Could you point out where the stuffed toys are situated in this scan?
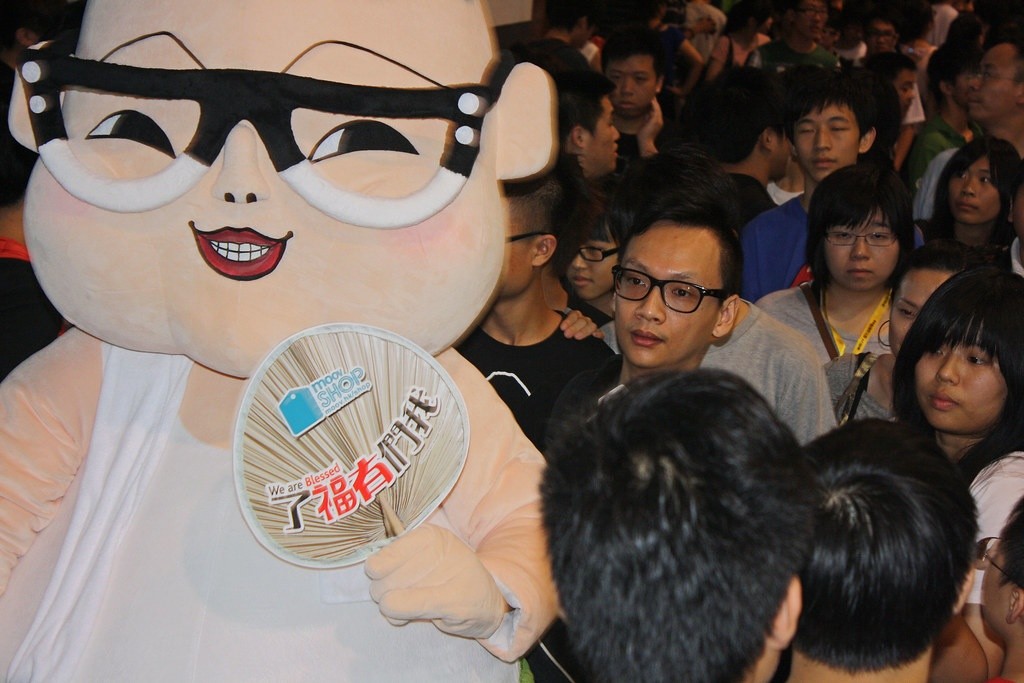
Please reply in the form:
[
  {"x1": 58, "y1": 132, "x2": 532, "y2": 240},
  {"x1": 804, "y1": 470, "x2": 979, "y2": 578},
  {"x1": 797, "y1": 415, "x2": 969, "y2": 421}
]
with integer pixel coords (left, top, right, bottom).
[{"x1": 0, "y1": 0, "x2": 562, "y2": 683}]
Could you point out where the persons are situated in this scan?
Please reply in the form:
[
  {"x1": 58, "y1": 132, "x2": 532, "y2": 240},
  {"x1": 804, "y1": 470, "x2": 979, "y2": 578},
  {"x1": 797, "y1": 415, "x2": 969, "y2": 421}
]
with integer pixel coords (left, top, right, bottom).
[
  {"x1": 455, "y1": 0, "x2": 1024, "y2": 683},
  {"x1": 0, "y1": 0, "x2": 88, "y2": 381}
]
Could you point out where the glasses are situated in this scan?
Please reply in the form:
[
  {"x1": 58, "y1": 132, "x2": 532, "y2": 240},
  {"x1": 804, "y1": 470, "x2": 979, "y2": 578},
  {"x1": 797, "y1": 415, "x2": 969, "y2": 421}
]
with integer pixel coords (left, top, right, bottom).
[
  {"x1": 968, "y1": 66, "x2": 1019, "y2": 82},
  {"x1": 564, "y1": 246, "x2": 619, "y2": 262},
  {"x1": 825, "y1": 231, "x2": 897, "y2": 247},
  {"x1": 611, "y1": 265, "x2": 725, "y2": 313},
  {"x1": 973, "y1": 536, "x2": 1018, "y2": 588},
  {"x1": 867, "y1": 29, "x2": 894, "y2": 40},
  {"x1": 794, "y1": 7, "x2": 828, "y2": 18}
]
